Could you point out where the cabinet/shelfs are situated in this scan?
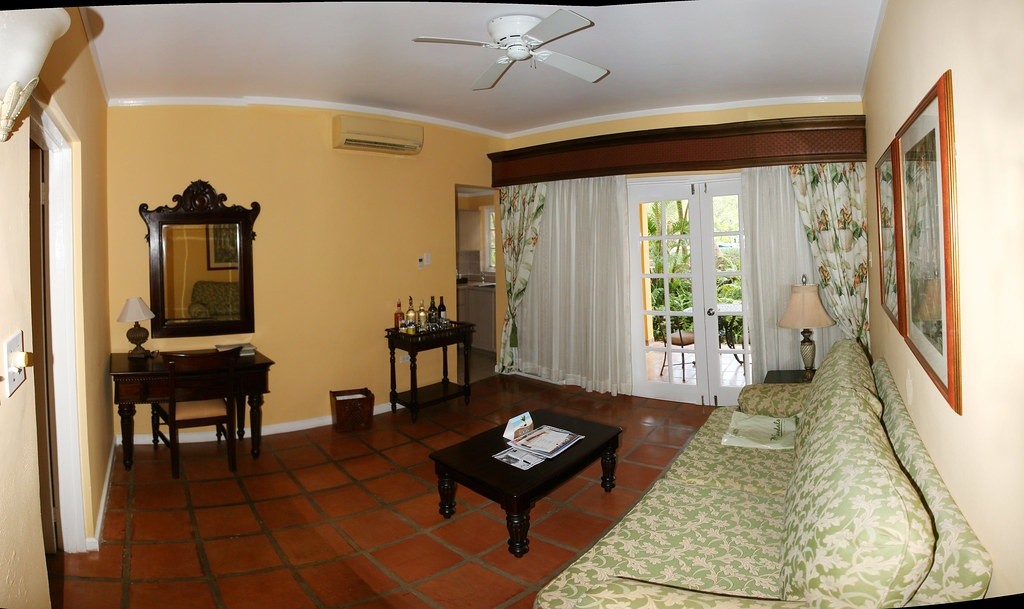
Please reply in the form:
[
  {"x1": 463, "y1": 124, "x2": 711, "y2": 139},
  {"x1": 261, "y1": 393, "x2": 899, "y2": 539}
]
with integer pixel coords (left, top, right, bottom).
[
  {"x1": 384, "y1": 319, "x2": 478, "y2": 424},
  {"x1": 456, "y1": 289, "x2": 495, "y2": 352}
]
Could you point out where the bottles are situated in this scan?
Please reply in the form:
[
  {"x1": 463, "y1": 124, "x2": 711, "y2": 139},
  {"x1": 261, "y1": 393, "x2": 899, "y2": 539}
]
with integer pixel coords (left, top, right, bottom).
[
  {"x1": 405, "y1": 295, "x2": 416, "y2": 326},
  {"x1": 400, "y1": 320, "x2": 407, "y2": 333},
  {"x1": 427, "y1": 296, "x2": 438, "y2": 324},
  {"x1": 407, "y1": 320, "x2": 415, "y2": 335},
  {"x1": 438, "y1": 296, "x2": 446, "y2": 322},
  {"x1": 416, "y1": 300, "x2": 427, "y2": 326},
  {"x1": 394, "y1": 299, "x2": 404, "y2": 328}
]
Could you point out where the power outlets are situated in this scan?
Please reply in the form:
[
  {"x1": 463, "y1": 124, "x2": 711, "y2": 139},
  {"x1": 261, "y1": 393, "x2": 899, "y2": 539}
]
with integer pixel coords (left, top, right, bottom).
[{"x1": 4, "y1": 328, "x2": 26, "y2": 399}]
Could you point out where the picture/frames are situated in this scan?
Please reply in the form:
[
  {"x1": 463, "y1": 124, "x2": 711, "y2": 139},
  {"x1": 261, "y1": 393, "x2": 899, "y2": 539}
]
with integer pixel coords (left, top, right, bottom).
[
  {"x1": 875, "y1": 69, "x2": 962, "y2": 416},
  {"x1": 206, "y1": 222, "x2": 240, "y2": 271}
]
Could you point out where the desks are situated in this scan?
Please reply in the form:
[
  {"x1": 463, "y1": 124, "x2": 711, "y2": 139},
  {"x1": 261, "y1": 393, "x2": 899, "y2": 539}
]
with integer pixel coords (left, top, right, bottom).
[
  {"x1": 109, "y1": 348, "x2": 276, "y2": 472},
  {"x1": 683, "y1": 302, "x2": 744, "y2": 365}
]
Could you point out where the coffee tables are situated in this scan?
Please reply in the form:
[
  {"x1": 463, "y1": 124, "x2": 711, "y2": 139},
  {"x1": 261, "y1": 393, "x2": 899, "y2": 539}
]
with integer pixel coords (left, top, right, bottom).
[
  {"x1": 427, "y1": 408, "x2": 624, "y2": 559},
  {"x1": 764, "y1": 368, "x2": 817, "y2": 382}
]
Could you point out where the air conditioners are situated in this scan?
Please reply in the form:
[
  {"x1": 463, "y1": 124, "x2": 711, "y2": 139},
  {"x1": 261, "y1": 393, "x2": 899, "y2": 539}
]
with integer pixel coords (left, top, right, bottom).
[{"x1": 330, "y1": 114, "x2": 424, "y2": 156}]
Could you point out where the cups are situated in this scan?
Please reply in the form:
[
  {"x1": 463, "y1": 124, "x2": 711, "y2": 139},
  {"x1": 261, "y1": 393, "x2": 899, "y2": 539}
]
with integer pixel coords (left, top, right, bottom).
[{"x1": 419, "y1": 319, "x2": 450, "y2": 334}]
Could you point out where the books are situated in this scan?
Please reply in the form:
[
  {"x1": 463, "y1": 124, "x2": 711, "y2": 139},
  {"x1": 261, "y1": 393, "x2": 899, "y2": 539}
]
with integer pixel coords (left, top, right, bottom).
[{"x1": 507, "y1": 425, "x2": 586, "y2": 459}]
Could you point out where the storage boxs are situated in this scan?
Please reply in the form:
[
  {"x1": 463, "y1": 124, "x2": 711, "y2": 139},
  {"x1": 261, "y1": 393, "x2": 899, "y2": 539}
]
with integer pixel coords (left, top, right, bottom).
[{"x1": 328, "y1": 385, "x2": 375, "y2": 433}]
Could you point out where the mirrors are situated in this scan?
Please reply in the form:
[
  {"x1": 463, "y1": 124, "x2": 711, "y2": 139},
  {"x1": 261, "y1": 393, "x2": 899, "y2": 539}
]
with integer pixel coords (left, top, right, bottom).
[{"x1": 138, "y1": 178, "x2": 262, "y2": 340}]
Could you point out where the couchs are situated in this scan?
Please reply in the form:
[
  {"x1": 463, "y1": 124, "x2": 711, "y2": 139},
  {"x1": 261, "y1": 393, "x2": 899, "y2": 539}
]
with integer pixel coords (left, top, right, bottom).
[
  {"x1": 188, "y1": 280, "x2": 241, "y2": 322},
  {"x1": 532, "y1": 336, "x2": 992, "y2": 609}
]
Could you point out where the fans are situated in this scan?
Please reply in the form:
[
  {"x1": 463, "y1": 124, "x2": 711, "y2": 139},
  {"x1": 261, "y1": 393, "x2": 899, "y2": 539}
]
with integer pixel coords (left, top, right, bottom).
[{"x1": 412, "y1": 7, "x2": 609, "y2": 91}]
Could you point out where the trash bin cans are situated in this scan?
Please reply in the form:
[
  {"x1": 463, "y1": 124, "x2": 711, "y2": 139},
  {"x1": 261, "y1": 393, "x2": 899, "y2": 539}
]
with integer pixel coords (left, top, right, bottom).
[{"x1": 330, "y1": 387, "x2": 376, "y2": 434}]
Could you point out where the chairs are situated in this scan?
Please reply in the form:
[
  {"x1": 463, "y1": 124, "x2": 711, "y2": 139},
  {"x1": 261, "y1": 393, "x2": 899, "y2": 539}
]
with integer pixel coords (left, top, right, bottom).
[
  {"x1": 150, "y1": 345, "x2": 246, "y2": 479},
  {"x1": 657, "y1": 314, "x2": 695, "y2": 382}
]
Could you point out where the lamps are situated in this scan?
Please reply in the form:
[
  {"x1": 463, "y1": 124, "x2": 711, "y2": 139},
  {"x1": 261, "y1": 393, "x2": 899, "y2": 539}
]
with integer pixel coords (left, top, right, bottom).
[
  {"x1": 117, "y1": 297, "x2": 155, "y2": 358},
  {"x1": 777, "y1": 274, "x2": 837, "y2": 381},
  {"x1": 1, "y1": 7, "x2": 73, "y2": 144}
]
